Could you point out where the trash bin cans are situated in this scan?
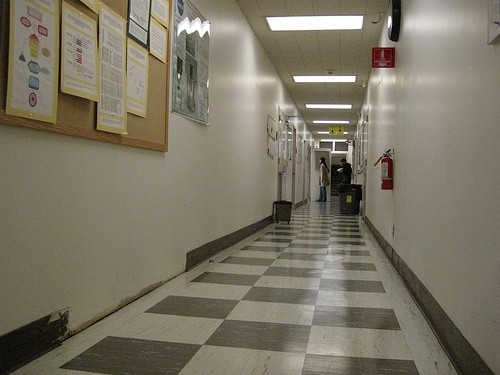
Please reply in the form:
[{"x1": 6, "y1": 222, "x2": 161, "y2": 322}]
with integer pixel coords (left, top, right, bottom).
[
  {"x1": 272, "y1": 200, "x2": 292, "y2": 225},
  {"x1": 338, "y1": 183, "x2": 363, "y2": 216}
]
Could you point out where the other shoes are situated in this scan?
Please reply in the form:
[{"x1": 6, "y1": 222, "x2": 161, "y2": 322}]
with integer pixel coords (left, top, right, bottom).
[
  {"x1": 322, "y1": 200, "x2": 326, "y2": 202},
  {"x1": 316, "y1": 199, "x2": 322, "y2": 201}
]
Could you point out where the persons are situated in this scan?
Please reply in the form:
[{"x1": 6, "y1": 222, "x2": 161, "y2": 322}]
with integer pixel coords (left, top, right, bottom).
[
  {"x1": 316, "y1": 156, "x2": 330, "y2": 202},
  {"x1": 339, "y1": 158, "x2": 352, "y2": 184}
]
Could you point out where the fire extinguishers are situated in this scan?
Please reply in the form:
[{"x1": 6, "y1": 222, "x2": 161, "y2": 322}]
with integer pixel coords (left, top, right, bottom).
[{"x1": 374, "y1": 149, "x2": 394, "y2": 191}]
